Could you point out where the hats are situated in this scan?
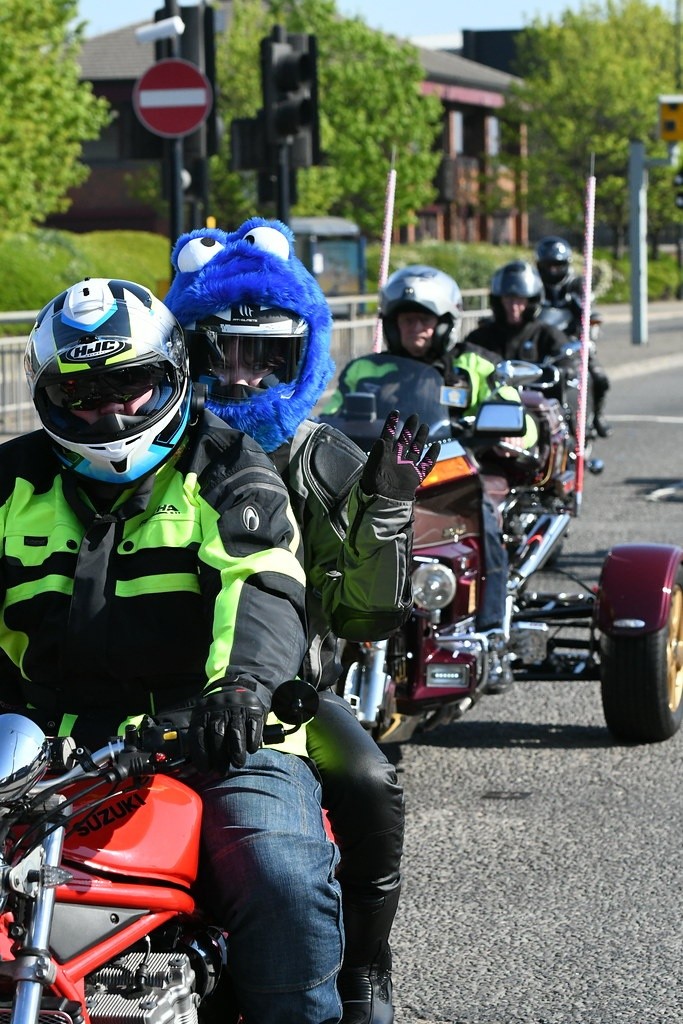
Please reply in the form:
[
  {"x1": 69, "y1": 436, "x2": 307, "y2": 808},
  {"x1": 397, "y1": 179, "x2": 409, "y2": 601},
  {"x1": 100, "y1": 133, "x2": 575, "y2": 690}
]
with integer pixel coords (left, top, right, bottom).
[{"x1": 162, "y1": 220, "x2": 334, "y2": 453}]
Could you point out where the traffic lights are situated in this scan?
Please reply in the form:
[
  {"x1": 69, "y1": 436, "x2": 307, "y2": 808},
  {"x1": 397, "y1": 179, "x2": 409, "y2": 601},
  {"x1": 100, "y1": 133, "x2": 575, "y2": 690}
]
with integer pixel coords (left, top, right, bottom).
[
  {"x1": 259, "y1": 21, "x2": 314, "y2": 149},
  {"x1": 659, "y1": 94, "x2": 682, "y2": 145}
]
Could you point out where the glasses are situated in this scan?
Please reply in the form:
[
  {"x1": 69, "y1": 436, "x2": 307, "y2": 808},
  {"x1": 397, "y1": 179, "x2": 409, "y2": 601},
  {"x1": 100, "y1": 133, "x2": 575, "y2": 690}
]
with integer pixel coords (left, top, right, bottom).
[{"x1": 59, "y1": 369, "x2": 161, "y2": 414}]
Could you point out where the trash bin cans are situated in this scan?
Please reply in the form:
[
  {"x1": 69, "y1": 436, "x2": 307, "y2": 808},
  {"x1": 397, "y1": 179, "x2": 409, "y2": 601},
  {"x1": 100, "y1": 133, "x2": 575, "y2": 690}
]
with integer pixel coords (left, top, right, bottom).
[{"x1": 292, "y1": 215, "x2": 365, "y2": 317}]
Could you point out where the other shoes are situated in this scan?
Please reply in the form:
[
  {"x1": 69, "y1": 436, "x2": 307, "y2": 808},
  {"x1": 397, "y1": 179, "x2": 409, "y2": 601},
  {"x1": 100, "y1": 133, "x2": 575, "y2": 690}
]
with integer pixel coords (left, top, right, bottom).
[
  {"x1": 349, "y1": 963, "x2": 394, "y2": 1024},
  {"x1": 593, "y1": 415, "x2": 612, "y2": 440}
]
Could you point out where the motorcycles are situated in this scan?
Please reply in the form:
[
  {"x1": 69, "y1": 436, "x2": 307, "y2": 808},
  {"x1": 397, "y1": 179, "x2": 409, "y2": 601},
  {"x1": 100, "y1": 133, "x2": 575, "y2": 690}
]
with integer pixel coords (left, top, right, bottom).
[
  {"x1": 0, "y1": 704, "x2": 339, "y2": 1023},
  {"x1": 308, "y1": 291, "x2": 683, "y2": 747}
]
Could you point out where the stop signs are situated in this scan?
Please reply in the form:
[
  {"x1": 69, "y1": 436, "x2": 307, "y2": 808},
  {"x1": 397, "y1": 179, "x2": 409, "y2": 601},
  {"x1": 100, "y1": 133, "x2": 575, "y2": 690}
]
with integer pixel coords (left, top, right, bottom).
[{"x1": 132, "y1": 58, "x2": 213, "y2": 139}]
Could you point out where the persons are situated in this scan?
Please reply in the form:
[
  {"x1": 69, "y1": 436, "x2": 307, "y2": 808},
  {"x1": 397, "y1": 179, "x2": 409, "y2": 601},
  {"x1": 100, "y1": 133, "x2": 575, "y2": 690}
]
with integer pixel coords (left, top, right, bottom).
[
  {"x1": 318, "y1": 264, "x2": 538, "y2": 697},
  {"x1": 532, "y1": 238, "x2": 611, "y2": 437},
  {"x1": 157, "y1": 219, "x2": 441, "y2": 1024},
  {"x1": 1, "y1": 276, "x2": 344, "y2": 1024},
  {"x1": 461, "y1": 260, "x2": 594, "y2": 475}
]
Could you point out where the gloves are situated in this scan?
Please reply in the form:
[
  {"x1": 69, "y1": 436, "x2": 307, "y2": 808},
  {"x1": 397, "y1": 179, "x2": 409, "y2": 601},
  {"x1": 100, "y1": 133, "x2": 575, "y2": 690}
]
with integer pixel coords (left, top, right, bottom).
[
  {"x1": 184, "y1": 685, "x2": 267, "y2": 774},
  {"x1": 358, "y1": 409, "x2": 442, "y2": 502}
]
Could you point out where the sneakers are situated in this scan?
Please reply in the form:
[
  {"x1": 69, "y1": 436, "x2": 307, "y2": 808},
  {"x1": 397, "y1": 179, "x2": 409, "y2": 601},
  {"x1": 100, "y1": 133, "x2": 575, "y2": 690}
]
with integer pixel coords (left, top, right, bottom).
[{"x1": 483, "y1": 634, "x2": 513, "y2": 692}]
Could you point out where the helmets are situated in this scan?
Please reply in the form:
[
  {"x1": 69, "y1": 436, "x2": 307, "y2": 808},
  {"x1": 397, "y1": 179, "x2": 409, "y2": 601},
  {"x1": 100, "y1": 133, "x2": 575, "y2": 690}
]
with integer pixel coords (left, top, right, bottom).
[
  {"x1": 538, "y1": 235, "x2": 572, "y2": 290},
  {"x1": 486, "y1": 264, "x2": 546, "y2": 324},
  {"x1": 27, "y1": 279, "x2": 193, "y2": 484},
  {"x1": 380, "y1": 266, "x2": 464, "y2": 358},
  {"x1": 185, "y1": 297, "x2": 310, "y2": 404}
]
